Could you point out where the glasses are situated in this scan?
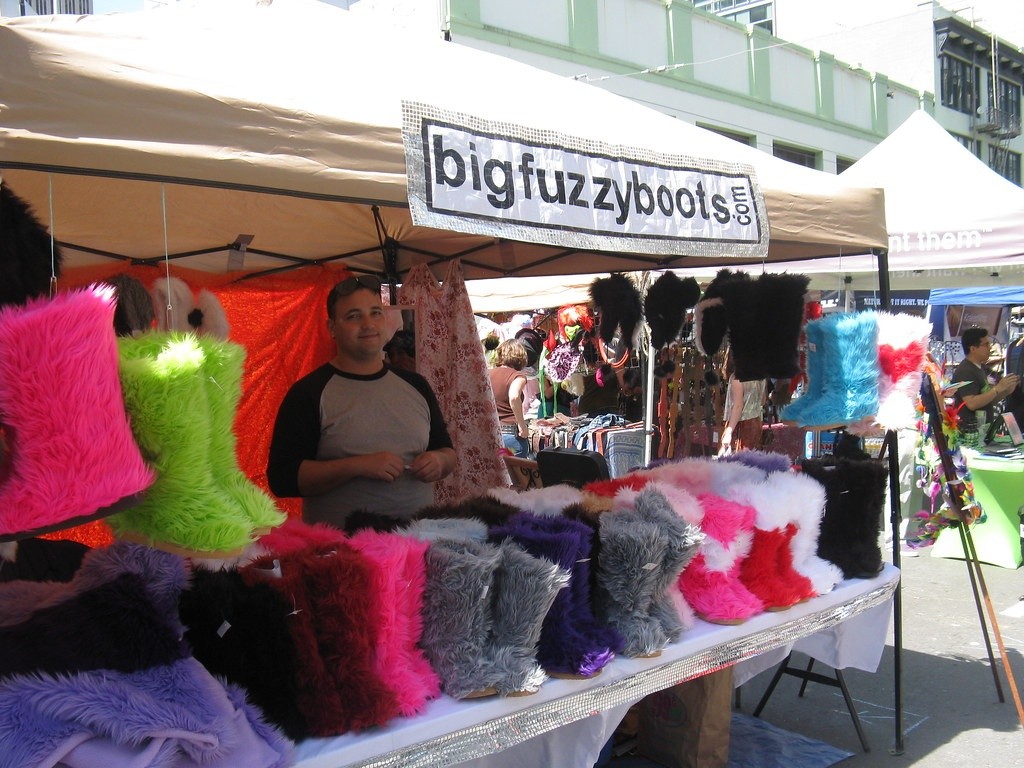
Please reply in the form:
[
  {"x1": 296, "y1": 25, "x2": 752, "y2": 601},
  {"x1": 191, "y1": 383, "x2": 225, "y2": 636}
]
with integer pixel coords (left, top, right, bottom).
[
  {"x1": 979, "y1": 341, "x2": 990, "y2": 348},
  {"x1": 327, "y1": 275, "x2": 381, "y2": 317}
]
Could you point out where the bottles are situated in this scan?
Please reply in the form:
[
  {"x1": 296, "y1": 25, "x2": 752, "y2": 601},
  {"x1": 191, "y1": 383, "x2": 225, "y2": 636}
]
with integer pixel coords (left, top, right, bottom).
[{"x1": 963, "y1": 418, "x2": 978, "y2": 448}]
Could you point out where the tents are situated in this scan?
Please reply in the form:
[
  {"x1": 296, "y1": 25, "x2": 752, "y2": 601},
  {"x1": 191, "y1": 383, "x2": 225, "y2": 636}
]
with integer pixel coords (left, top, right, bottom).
[{"x1": 0, "y1": 1, "x2": 1024, "y2": 768}]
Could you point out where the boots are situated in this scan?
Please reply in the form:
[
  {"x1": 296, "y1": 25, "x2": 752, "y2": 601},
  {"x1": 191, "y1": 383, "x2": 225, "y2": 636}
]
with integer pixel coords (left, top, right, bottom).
[
  {"x1": 874, "y1": 309, "x2": 935, "y2": 432},
  {"x1": 0, "y1": 280, "x2": 157, "y2": 541},
  {"x1": 779, "y1": 312, "x2": 881, "y2": 427},
  {"x1": 98, "y1": 331, "x2": 287, "y2": 551},
  {"x1": 189, "y1": 449, "x2": 890, "y2": 735}
]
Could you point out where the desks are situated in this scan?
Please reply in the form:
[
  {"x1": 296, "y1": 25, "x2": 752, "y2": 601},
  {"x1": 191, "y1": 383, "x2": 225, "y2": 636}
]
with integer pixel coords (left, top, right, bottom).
[
  {"x1": 291, "y1": 560, "x2": 901, "y2": 768},
  {"x1": 930, "y1": 435, "x2": 1024, "y2": 570},
  {"x1": 526, "y1": 424, "x2": 654, "y2": 479}
]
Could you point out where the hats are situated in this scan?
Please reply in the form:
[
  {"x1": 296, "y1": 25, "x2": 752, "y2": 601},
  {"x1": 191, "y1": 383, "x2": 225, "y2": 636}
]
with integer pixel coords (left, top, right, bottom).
[
  {"x1": 546, "y1": 342, "x2": 582, "y2": 383},
  {"x1": 598, "y1": 326, "x2": 630, "y2": 368},
  {"x1": 596, "y1": 368, "x2": 605, "y2": 388},
  {"x1": 514, "y1": 328, "x2": 543, "y2": 367}
]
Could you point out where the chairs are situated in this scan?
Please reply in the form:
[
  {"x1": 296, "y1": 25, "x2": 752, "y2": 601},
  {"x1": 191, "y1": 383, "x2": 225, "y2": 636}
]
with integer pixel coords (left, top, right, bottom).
[{"x1": 537, "y1": 446, "x2": 610, "y2": 490}]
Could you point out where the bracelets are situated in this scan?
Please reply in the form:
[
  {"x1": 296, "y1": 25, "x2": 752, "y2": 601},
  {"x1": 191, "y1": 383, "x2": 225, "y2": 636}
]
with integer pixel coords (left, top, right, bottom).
[{"x1": 993, "y1": 385, "x2": 999, "y2": 397}]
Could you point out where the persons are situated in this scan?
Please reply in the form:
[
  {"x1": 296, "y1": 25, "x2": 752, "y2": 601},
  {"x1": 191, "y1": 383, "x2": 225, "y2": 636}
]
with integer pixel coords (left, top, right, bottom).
[
  {"x1": 486, "y1": 340, "x2": 529, "y2": 459},
  {"x1": 951, "y1": 328, "x2": 1021, "y2": 440},
  {"x1": 721, "y1": 371, "x2": 766, "y2": 453},
  {"x1": 267, "y1": 276, "x2": 459, "y2": 532},
  {"x1": 578, "y1": 371, "x2": 619, "y2": 415},
  {"x1": 537, "y1": 378, "x2": 572, "y2": 419}
]
